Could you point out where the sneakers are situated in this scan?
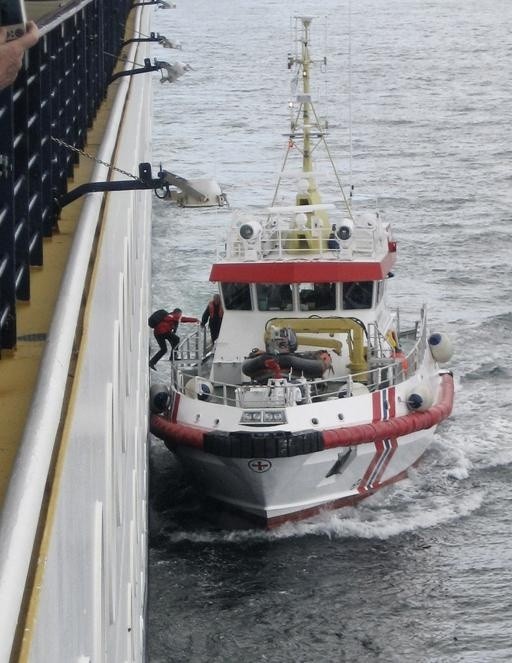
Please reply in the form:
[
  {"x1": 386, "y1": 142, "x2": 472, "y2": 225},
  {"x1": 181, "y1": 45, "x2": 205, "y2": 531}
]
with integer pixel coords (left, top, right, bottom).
[
  {"x1": 149, "y1": 361, "x2": 156, "y2": 372},
  {"x1": 169, "y1": 357, "x2": 181, "y2": 362}
]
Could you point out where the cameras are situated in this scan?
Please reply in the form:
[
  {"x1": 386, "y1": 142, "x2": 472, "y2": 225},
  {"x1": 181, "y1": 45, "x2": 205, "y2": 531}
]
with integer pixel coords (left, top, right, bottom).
[{"x1": 0, "y1": 0, "x2": 28, "y2": 41}]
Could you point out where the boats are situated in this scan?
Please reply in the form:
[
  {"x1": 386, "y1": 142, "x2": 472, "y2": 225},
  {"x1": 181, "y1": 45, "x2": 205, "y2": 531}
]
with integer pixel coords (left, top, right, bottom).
[{"x1": 149, "y1": 14, "x2": 455, "y2": 531}]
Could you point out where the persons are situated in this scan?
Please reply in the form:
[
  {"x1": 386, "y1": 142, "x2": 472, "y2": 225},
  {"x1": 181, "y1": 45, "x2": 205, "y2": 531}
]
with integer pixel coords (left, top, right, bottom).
[
  {"x1": 1, "y1": 19, "x2": 40, "y2": 91},
  {"x1": 148, "y1": 307, "x2": 200, "y2": 370},
  {"x1": 201, "y1": 293, "x2": 224, "y2": 344}
]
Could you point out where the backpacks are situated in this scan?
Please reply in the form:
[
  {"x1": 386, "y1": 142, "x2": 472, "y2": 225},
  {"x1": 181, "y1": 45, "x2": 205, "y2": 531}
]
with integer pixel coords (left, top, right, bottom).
[{"x1": 148, "y1": 308, "x2": 168, "y2": 329}]
